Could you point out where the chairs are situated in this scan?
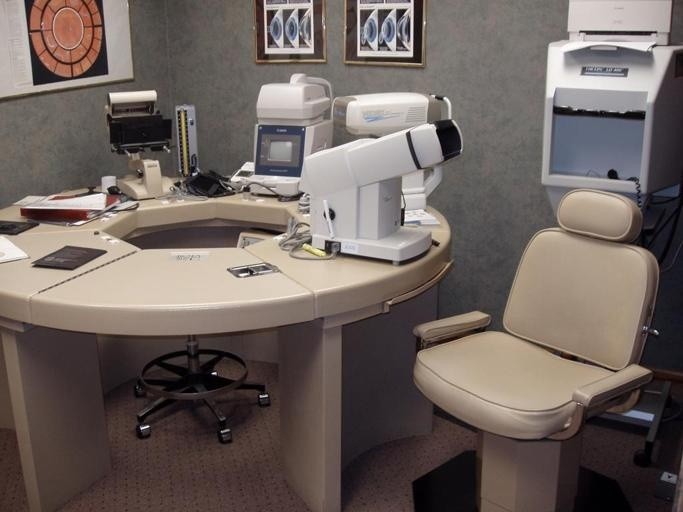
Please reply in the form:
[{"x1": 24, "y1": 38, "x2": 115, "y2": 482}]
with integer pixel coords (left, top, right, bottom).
[{"x1": 413, "y1": 188, "x2": 660, "y2": 512}]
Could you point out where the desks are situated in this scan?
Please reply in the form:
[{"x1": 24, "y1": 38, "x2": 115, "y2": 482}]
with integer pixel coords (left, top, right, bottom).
[{"x1": 0, "y1": 178, "x2": 454, "y2": 512}]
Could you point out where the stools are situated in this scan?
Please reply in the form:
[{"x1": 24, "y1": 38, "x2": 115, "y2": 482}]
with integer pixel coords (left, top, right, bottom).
[{"x1": 130, "y1": 335, "x2": 271, "y2": 444}]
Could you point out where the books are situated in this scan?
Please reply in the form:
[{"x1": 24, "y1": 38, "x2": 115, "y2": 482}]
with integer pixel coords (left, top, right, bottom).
[
  {"x1": 402, "y1": 207, "x2": 441, "y2": 225},
  {"x1": 19, "y1": 194, "x2": 122, "y2": 220}
]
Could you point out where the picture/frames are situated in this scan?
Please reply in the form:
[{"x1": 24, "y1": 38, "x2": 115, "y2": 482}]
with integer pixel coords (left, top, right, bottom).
[
  {"x1": 342, "y1": 0, "x2": 425, "y2": 69},
  {"x1": 254, "y1": 0, "x2": 327, "y2": 65}
]
